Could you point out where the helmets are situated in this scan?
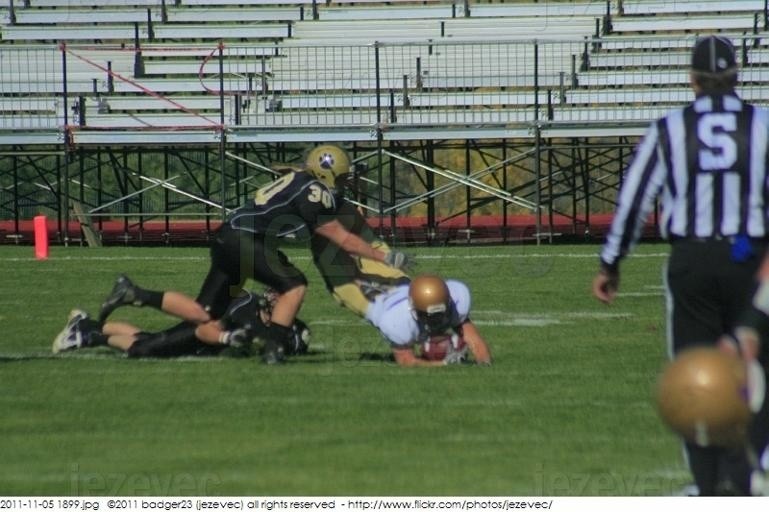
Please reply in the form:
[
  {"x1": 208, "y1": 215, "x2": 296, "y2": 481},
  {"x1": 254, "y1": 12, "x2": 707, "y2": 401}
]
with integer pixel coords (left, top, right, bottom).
[
  {"x1": 304, "y1": 145, "x2": 358, "y2": 201},
  {"x1": 258, "y1": 286, "x2": 279, "y2": 314},
  {"x1": 654, "y1": 344, "x2": 751, "y2": 445},
  {"x1": 409, "y1": 275, "x2": 453, "y2": 335}
]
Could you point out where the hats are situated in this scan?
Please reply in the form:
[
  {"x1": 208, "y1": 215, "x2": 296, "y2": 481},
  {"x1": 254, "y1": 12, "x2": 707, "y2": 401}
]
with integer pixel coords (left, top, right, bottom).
[{"x1": 692, "y1": 37, "x2": 737, "y2": 79}]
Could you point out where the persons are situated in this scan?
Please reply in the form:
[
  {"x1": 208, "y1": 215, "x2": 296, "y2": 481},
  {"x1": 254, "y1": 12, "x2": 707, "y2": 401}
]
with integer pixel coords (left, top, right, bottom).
[
  {"x1": 52, "y1": 271, "x2": 312, "y2": 358},
  {"x1": 656, "y1": 247, "x2": 768, "y2": 495},
  {"x1": 592, "y1": 36, "x2": 768, "y2": 494},
  {"x1": 272, "y1": 158, "x2": 493, "y2": 370},
  {"x1": 99, "y1": 145, "x2": 422, "y2": 365}
]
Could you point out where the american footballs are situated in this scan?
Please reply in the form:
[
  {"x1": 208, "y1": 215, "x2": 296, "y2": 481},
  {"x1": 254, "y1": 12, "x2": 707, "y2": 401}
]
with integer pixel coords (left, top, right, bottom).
[{"x1": 420, "y1": 332, "x2": 469, "y2": 362}]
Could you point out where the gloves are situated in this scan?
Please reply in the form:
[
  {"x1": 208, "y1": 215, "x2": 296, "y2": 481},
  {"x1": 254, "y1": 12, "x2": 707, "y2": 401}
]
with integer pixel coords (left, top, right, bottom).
[
  {"x1": 227, "y1": 329, "x2": 251, "y2": 350},
  {"x1": 444, "y1": 342, "x2": 469, "y2": 365},
  {"x1": 384, "y1": 251, "x2": 418, "y2": 274}
]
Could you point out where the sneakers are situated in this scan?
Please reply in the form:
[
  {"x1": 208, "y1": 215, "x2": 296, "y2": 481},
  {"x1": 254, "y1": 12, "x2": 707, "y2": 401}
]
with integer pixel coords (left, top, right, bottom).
[
  {"x1": 270, "y1": 162, "x2": 305, "y2": 179},
  {"x1": 52, "y1": 274, "x2": 132, "y2": 355},
  {"x1": 263, "y1": 338, "x2": 286, "y2": 365}
]
